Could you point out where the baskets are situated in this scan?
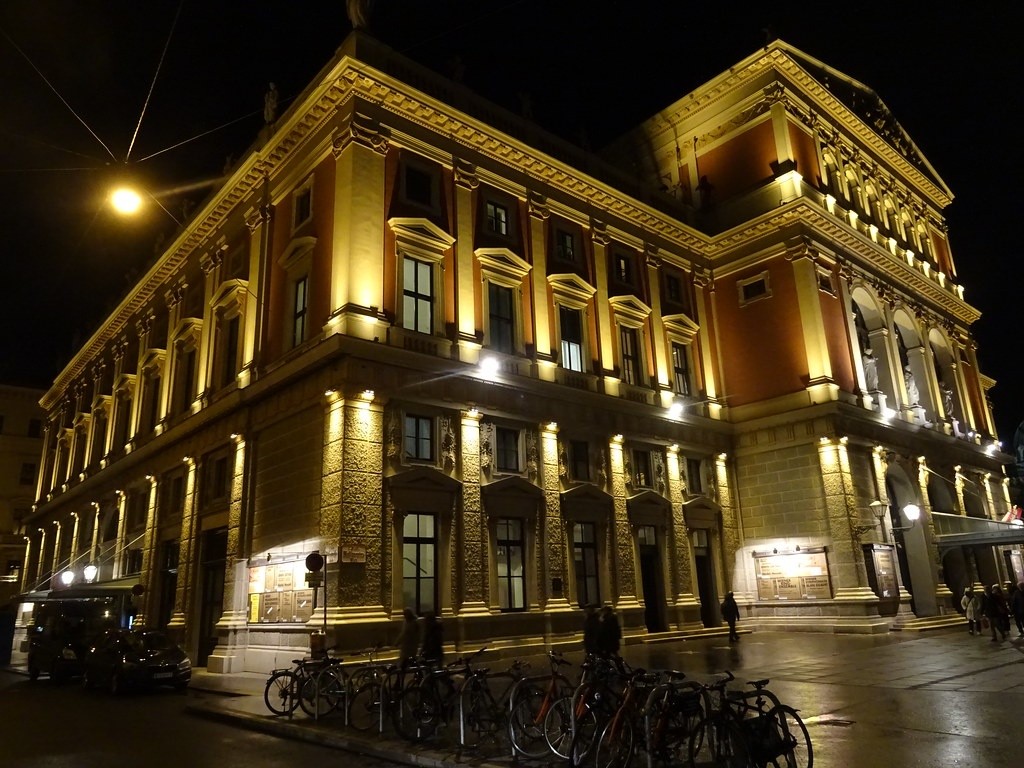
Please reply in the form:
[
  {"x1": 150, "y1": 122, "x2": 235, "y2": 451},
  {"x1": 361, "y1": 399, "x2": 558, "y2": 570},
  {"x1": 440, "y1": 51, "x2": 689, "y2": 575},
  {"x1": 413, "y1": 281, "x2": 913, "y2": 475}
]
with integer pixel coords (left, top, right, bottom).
[{"x1": 739, "y1": 712, "x2": 784, "y2": 764}]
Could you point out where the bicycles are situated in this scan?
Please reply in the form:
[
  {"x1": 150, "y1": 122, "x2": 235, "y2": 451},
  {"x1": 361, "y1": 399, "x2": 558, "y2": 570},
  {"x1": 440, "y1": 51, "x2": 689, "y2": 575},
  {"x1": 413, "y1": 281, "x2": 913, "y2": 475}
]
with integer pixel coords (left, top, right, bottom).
[{"x1": 264, "y1": 642, "x2": 814, "y2": 768}]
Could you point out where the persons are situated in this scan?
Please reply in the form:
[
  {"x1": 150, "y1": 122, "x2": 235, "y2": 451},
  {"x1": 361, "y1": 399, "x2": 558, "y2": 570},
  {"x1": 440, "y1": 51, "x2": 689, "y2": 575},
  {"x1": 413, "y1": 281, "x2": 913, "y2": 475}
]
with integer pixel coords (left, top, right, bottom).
[
  {"x1": 992, "y1": 586, "x2": 1011, "y2": 635},
  {"x1": 394, "y1": 607, "x2": 423, "y2": 679},
  {"x1": 581, "y1": 603, "x2": 601, "y2": 658},
  {"x1": 1005, "y1": 583, "x2": 1024, "y2": 637},
  {"x1": 419, "y1": 609, "x2": 443, "y2": 666},
  {"x1": 960, "y1": 587, "x2": 982, "y2": 635},
  {"x1": 596, "y1": 606, "x2": 624, "y2": 664},
  {"x1": 980, "y1": 585, "x2": 1006, "y2": 641},
  {"x1": 721, "y1": 591, "x2": 740, "y2": 642}
]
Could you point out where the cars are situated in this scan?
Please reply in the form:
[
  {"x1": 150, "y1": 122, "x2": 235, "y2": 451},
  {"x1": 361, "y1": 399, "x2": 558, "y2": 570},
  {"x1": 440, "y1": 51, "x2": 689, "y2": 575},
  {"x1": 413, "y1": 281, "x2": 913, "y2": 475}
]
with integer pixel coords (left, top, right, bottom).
[{"x1": 84, "y1": 629, "x2": 193, "y2": 695}]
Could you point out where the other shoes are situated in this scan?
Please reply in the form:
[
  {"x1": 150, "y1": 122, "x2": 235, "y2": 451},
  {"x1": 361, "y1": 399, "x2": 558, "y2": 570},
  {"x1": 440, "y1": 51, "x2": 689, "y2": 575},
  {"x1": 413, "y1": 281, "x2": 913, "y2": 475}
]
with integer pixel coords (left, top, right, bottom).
[
  {"x1": 735, "y1": 636, "x2": 740, "y2": 640},
  {"x1": 969, "y1": 630, "x2": 974, "y2": 635},
  {"x1": 1017, "y1": 633, "x2": 1024, "y2": 637},
  {"x1": 1002, "y1": 635, "x2": 1006, "y2": 641},
  {"x1": 976, "y1": 631, "x2": 982, "y2": 636},
  {"x1": 991, "y1": 638, "x2": 998, "y2": 641},
  {"x1": 729, "y1": 639, "x2": 735, "y2": 642}
]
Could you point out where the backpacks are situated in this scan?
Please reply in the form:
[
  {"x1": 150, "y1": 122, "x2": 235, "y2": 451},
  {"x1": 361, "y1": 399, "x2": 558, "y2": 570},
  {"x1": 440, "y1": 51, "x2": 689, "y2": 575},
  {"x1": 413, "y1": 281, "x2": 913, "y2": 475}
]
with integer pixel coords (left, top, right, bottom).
[{"x1": 720, "y1": 602, "x2": 728, "y2": 615}]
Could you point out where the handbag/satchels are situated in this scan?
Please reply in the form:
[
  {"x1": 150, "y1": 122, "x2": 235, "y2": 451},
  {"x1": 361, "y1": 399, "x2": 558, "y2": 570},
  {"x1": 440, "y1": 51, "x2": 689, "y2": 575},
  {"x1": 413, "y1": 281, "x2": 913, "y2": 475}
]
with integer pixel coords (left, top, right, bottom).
[
  {"x1": 982, "y1": 618, "x2": 990, "y2": 629},
  {"x1": 1003, "y1": 622, "x2": 1010, "y2": 631}
]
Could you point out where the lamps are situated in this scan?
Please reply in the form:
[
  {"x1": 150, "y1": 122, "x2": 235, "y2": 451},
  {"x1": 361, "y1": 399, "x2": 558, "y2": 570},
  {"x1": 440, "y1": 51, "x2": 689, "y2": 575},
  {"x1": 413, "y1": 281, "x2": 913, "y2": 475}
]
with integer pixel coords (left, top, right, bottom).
[
  {"x1": 62, "y1": 539, "x2": 122, "y2": 586},
  {"x1": 83, "y1": 535, "x2": 145, "y2": 583},
  {"x1": 869, "y1": 501, "x2": 887, "y2": 525},
  {"x1": 22, "y1": 603, "x2": 34, "y2": 613},
  {"x1": 903, "y1": 502, "x2": 921, "y2": 527}
]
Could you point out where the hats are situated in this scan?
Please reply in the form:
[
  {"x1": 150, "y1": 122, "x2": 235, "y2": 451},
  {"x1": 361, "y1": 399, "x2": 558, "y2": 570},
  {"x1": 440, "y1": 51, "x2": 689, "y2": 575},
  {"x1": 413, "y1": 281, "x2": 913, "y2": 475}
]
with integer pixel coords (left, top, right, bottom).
[
  {"x1": 727, "y1": 591, "x2": 734, "y2": 596},
  {"x1": 599, "y1": 607, "x2": 612, "y2": 611},
  {"x1": 963, "y1": 587, "x2": 972, "y2": 594},
  {"x1": 584, "y1": 603, "x2": 593, "y2": 606}
]
